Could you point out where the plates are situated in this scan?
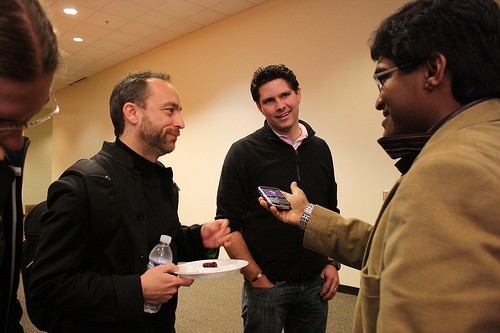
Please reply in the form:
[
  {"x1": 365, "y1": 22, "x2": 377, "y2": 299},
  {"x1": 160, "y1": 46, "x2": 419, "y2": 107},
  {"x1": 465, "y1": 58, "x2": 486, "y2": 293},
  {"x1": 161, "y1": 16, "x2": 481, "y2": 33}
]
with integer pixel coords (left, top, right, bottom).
[{"x1": 171, "y1": 258, "x2": 250, "y2": 280}]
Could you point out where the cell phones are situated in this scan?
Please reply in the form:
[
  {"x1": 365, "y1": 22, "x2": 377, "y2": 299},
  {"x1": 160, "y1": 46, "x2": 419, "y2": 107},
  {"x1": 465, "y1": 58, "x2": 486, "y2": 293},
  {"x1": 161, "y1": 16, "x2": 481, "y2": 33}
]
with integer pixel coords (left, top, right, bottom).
[{"x1": 258, "y1": 186, "x2": 292, "y2": 210}]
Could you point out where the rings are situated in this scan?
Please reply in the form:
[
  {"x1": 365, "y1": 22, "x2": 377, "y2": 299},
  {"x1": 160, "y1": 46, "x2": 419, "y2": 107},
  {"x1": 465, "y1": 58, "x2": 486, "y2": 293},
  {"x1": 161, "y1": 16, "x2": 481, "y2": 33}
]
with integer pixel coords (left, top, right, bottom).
[{"x1": 334, "y1": 288, "x2": 337, "y2": 291}]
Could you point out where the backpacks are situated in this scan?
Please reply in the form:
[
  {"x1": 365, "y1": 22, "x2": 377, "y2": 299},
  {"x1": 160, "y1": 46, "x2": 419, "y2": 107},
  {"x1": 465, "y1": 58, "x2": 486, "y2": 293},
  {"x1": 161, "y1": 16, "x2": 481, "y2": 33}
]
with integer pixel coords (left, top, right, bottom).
[{"x1": 22, "y1": 161, "x2": 179, "y2": 332}]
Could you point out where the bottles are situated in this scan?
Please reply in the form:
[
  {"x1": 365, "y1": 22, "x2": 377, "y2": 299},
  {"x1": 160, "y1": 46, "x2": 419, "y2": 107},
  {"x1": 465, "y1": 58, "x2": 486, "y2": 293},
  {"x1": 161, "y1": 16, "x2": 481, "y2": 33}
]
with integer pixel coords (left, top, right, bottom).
[{"x1": 143, "y1": 235, "x2": 173, "y2": 314}]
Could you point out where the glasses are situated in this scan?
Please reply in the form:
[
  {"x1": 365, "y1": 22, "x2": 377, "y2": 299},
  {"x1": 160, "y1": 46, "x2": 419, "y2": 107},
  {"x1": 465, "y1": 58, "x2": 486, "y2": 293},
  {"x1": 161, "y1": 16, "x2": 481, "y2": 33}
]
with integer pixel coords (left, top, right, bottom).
[
  {"x1": 0, "y1": 90, "x2": 60, "y2": 135},
  {"x1": 373, "y1": 57, "x2": 422, "y2": 91}
]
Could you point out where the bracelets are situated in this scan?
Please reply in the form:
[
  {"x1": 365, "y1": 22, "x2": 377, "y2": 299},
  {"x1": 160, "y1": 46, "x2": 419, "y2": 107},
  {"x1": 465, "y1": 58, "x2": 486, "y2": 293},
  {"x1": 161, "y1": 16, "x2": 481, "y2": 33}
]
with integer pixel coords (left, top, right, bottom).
[
  {"x1": 299, "y1": 203, "x2": 315, "y2": 230},
  {"x1": 250, "y1": 272, "x2": 264, "y2": 283}
]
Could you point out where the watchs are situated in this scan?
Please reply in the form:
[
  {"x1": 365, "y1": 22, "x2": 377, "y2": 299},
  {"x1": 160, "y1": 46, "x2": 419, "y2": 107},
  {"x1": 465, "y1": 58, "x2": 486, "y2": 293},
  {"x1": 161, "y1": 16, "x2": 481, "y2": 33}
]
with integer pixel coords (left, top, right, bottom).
[{"x1": 328, "y1": 260, "x2": 341, "y2": 271}]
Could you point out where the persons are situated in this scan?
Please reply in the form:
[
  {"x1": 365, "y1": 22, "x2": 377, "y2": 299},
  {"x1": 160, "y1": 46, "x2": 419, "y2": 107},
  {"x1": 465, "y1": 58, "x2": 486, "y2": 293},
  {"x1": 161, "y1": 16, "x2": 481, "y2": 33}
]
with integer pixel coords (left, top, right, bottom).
[
  {"x1": 214, "y1": 63, "x2": 341, "y2": 333},
  {"x1": 259, "y1": 1, "x2": 500, "y2": 333},
  {"x1": 0, "y1": 0, "x2": 59, "y2": 333},
  {"x1": 25, "y1": 71, "x2": 231, "y2": 333}
]
